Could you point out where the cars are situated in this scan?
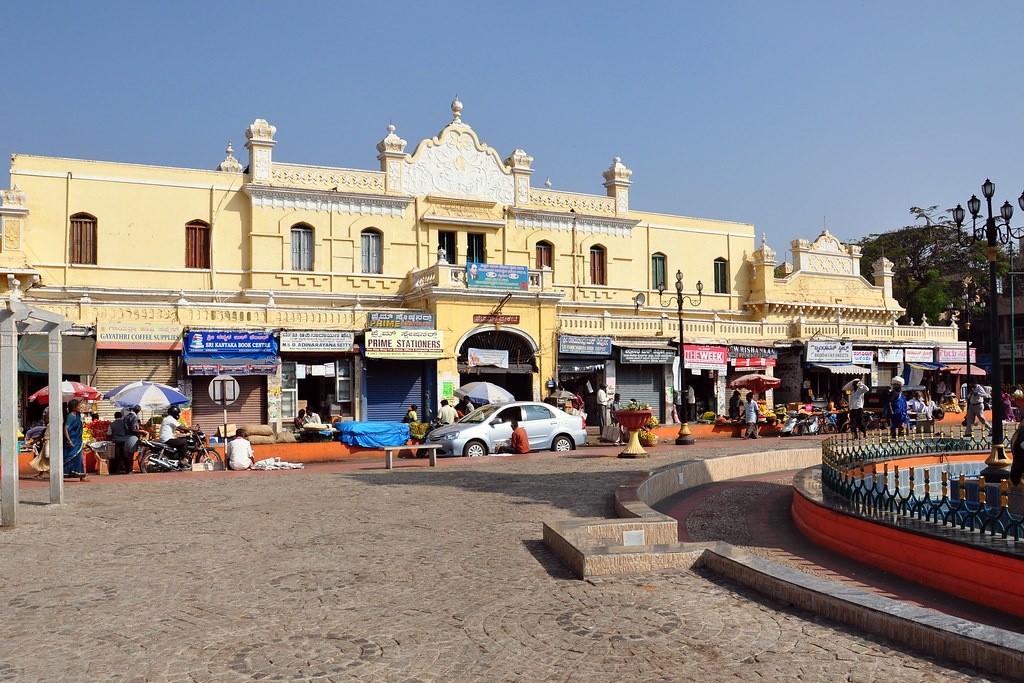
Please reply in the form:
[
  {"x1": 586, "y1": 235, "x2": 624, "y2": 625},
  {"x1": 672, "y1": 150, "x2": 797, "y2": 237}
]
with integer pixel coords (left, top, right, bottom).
[{"x1": 424, "y1": 399, "x2": 586, "y2": 460}]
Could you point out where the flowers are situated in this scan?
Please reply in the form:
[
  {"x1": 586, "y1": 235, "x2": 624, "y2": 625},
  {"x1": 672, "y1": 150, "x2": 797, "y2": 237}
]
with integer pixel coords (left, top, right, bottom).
[
  {"x1": 641, "y1": 432, "x2": 656, "y2": 439},
  {"x1": 622, "y1": 401, "x2": 652, "y2": 411},
  {"x1": 647, "y1": 416, "x2": 661, "y2": 428}
]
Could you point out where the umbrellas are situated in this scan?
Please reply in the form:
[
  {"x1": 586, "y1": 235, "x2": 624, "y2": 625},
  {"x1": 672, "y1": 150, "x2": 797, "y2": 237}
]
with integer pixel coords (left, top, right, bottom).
[
  {"x1": 28, "y1": 380, "x2": 101, "y2": 408},
  {"x1": 550, "y1": 388, "x2": 578, "y2": 399},
  {"x1": 453, "y1": 381, "x2": 515, "y2": 404},
  {"x1": 729, "y1": 374, "x2": 782, "y2": 397},
  {"x1": 103, "y1": 378, "x2": 190, "y2": 426}
]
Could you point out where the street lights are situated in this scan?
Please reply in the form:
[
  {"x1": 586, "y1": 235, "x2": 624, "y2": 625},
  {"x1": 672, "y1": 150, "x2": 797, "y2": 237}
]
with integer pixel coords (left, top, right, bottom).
[
  {"x1": 953, "y1": 179, "x2": 1024, "y2": 482},
  {"x1": 657, "y1": 269, "x2": 703, "y2": 445},
  {"x1": 947, "y1": 284, "x2": 987, "y2": 426}
]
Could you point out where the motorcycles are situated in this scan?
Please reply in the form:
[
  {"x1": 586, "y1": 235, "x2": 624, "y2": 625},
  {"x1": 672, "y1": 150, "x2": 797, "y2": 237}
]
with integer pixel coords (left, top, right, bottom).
[{"x1": 136, "y1": 423, "x2": 221, "y2": 473}]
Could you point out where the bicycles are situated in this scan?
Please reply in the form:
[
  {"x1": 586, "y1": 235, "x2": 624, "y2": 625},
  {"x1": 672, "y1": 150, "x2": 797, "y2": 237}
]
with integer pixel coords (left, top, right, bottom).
[{"x1": 839, "y1": 412, "x2": 873, "y2": 433}]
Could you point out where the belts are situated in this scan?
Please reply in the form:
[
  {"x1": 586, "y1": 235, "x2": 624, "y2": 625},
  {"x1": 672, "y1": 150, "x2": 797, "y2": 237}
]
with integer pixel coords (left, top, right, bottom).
[{"x1": 972, "y1": 403, "x2": 981, "y2": 405}]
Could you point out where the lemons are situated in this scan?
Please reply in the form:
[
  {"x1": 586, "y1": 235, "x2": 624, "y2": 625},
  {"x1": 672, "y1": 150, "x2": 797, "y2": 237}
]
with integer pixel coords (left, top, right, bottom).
[{"x1": 82, "y1": 428, "x2": 93, "y2": 440}]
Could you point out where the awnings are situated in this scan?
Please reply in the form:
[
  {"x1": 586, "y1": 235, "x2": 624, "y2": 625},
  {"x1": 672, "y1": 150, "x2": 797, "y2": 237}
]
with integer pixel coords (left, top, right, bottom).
[
  {"x1": 183, "y1": 354, "x2": 282, "y2": 376},
  {"x1": 807, "y1": 362, "x2": 870, "y2": 375},
  {"x1": 907, "y1": 363, "x2": 986, "y2": 376}
]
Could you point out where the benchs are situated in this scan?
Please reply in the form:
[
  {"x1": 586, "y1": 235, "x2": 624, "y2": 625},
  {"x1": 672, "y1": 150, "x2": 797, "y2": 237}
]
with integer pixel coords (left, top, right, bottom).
[{"x1": 379, "y1": 444, "x2": 443, "y2": 469}]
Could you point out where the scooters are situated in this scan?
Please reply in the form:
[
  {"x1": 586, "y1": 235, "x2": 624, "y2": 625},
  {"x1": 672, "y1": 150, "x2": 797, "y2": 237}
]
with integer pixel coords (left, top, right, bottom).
[{"x1": 778, "y1": 409, "x2": 823, "y2": 437}]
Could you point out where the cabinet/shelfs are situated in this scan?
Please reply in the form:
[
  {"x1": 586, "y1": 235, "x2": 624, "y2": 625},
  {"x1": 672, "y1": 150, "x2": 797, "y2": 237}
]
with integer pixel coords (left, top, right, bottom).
[
  {"x1": 282, "y1": 362, "x2": 298, "y2": 419},
  {"x1": 334, "y1": 359, "x2": 354, "y2": 402}
]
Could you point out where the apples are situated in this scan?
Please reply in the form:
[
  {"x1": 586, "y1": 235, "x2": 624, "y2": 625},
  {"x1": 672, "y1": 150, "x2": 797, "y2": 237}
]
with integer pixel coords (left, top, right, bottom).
[{"x1": 85, "y1": 419, "x2": 113, "y2": 441}]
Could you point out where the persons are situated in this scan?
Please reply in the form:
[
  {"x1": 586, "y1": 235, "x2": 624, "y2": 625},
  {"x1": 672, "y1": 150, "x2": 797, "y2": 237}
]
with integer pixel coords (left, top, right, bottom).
[
  {"x1": 159, "y1": 406, "x2": 198, "y2": 464},
  {"x1": 565, "y1": 391, "x2": 584, "y2": 415},
  {"x1": 850, "y1": 380, "x2": 870, "y2": 440},
  {"x1": 405, "y1": 404, "x2": 418, "y2": 423},
  {"x1": 105, "y1": 406, "x2": 148, "y2": 475},
  {"x1": 597, "y1": 383, "x2": 622, "y2": 435},
  {"x1": 686, "y1": 385, "x2": 696, "y2": 422},
  {"x1": 434, "y1": 400, "x2": 465, "y2": 427},
  {"x1": 464, "y1": 396, "x2": 475, "y2": 415},
  {"x1": 728, "y1": 391, "x2": 763, "y2": 440},
  {"x1": 510, "y1": 420, "x2": 531, "y2": 454},
  {"x1": 226, "y1": 429, "x2": 254, "y2": 471},
  {"x1": 881, "y1": 375, "x2": 937, "y2": 439},
  {"x1": 1001, "y1": 384, "x2": 1024, "y2": 422},
  {"x1": 35, "y1": 399, "x2": 91, "y2": 482},
  {"x1": 963, "y1": 381, "x2": 992, "y2": 437},
  {"x1": 91, "y1": 414, "x2": 99, "y2": 421},
  {"x1": 294, "y1": 406, "x2": 322, "y2": 442}
]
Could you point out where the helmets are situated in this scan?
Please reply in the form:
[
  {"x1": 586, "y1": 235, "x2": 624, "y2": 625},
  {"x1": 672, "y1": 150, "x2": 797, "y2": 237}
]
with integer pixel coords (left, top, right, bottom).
[{"x1": 168, "y1": 406, "x2": 180, "y2": 420}]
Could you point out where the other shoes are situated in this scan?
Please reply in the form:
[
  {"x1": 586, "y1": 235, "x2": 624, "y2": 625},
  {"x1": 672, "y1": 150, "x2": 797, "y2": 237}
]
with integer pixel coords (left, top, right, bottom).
[
  {"x1": 988, "y1": 434, "x2": 992, "y2": 436},
  {"x1": 180, "y1": 461, "x2": 191, "y2": 467},
  {"x1": 963, "y1": 434, "x2": 972, "y2": 437}
]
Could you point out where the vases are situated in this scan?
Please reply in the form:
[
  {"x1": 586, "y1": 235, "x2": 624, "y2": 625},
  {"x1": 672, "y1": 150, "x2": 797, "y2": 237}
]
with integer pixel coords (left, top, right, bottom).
[
  {"x1": 642, "y1": 439, "x2": 658, "y2": 447},
  {"x1": 610, "y1": 409, "x2": 657, "y2": 430}
]
